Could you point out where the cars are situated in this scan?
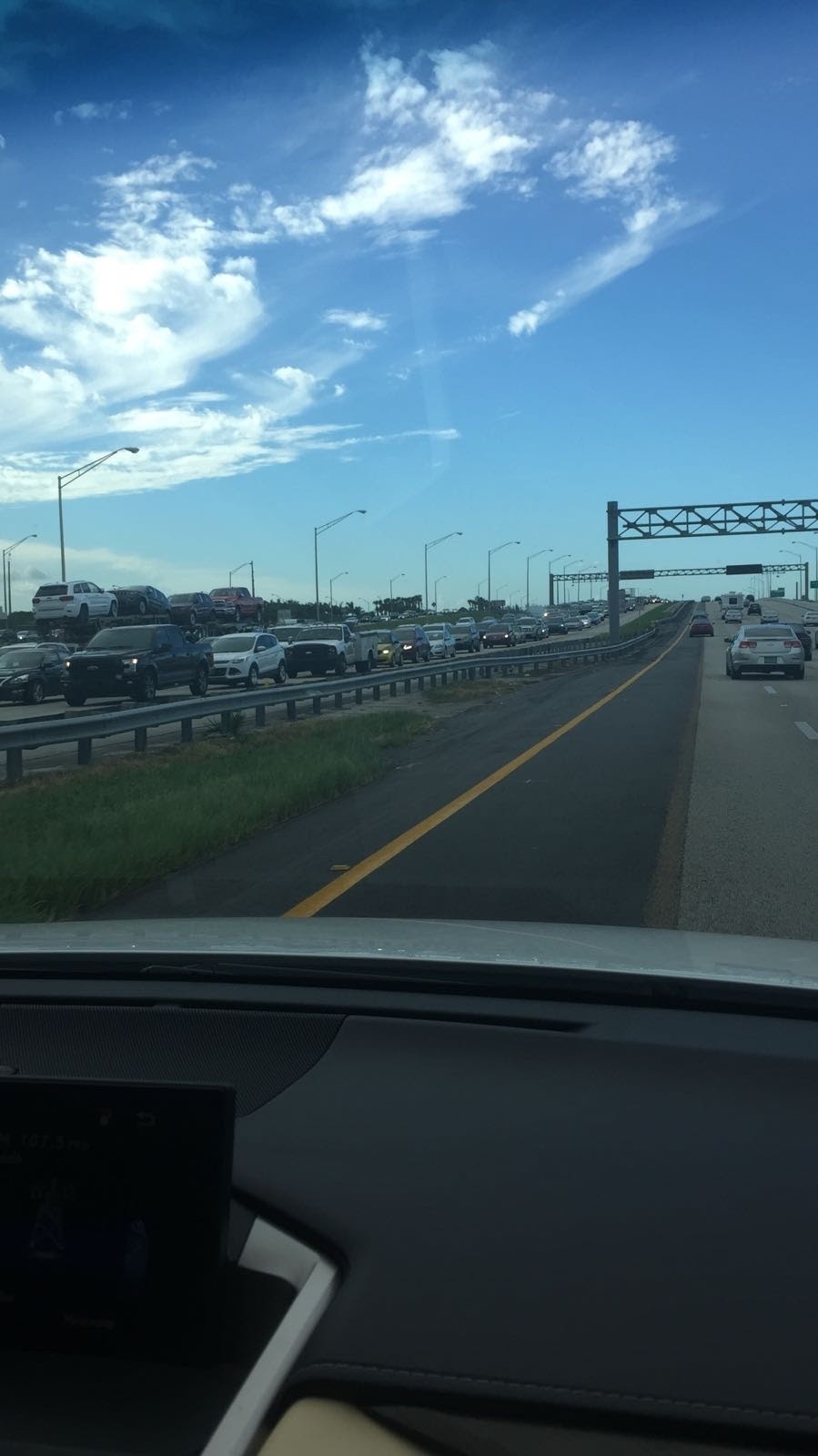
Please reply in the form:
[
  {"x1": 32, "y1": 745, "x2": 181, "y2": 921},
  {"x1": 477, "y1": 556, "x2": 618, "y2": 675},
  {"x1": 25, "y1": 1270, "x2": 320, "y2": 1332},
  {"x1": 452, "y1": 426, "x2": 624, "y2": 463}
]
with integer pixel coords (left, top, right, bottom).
[
  {"x1": 691, "y1": 611, "x2": 709, "y2": 622},
  {"x1": 765, "y1": 621, "x2": 813, "y2": 661},
  {"x1": 760, "y1": 610, "x2": 779, "y2": 624},
  {"x1": 801, "y1": 611, "x2": 818, "y2": 627},
  {"x1": 723, "y1": 624, "x2": 805, "y2": 680},
  {"x1": 700, "y1": 591, "x2": 762, "y2": 624},
  {"x1": 0, "y1": 576, "x2": 671, "y2": 710},
  {"x1": 689, "y1": 618, "x2": 716, "y2": 638}
]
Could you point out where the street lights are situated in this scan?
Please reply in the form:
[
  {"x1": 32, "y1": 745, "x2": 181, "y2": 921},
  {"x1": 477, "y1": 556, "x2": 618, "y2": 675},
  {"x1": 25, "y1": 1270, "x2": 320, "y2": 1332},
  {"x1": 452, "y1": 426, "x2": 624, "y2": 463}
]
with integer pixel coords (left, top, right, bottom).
[
  {"x1": 487, "y1": 541, "x2": 520, "y2": 616},
  {"x1": 549, "y1": 554, "x2": 571, "y2": 606},
  {"x1": 779, "y1": 550, "x2": 802, "y2": 600},
  {"x1": 563, "y1": 560, "x2": 583, "y2": 604},
  {"x1": 390, "y1": 573, "x2": 406, "y2": 619},
  {"x1": 526, "y1": 548, "x2": 554, "y2": 608},
  {"x1": 314, "y1": 509, "x2": 367, "y2": 621},
  {"x1": 424, "y1": 531, "x2": 462, "y2": 615},
  {"x1": 56, "y1": 447, "x2": 140, "y2": 581},
  {"x1": 228, "y1": 562, "x2": 253, "y2": 588},
  {"x1": 791, "y1": 541, "x2": 818, "y2": 601},
  {"x1": 434, "y1": 576, "x2": 448, "y2": 615},
  {"x1": 330, "y1": 571, "x2": 349, "y2": 623},
  {"x1": 577, "y1": 565, "x2": 597, "y2": 602},
  {"x1": 2, "y1": 534, "x2": 37, "y2": 629}
]
